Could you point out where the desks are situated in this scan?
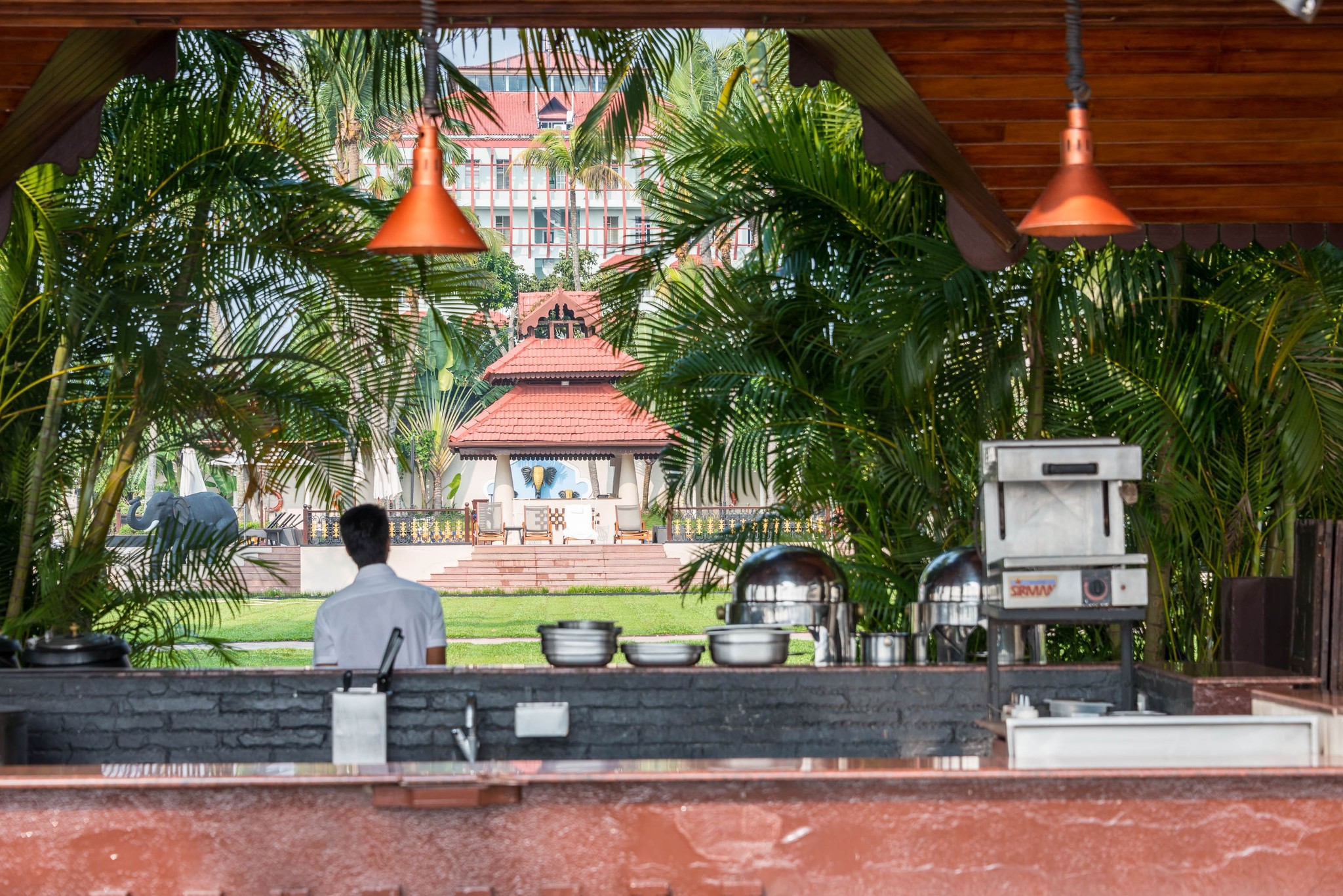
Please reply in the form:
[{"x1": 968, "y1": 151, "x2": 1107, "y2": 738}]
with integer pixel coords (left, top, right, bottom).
[{"x1": 503, "y1": 526, "x2": 524, "y2": 545}]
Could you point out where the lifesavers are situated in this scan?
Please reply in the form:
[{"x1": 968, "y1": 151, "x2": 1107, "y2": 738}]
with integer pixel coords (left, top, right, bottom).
[
  {"x1": 269, "y1": 490, "x2": 284, "y2": 513},
  {"x1": 332, "y1": 489, "x2": 343, "y2": 510},
  {"x1": 729, "y1": 489, "x2": 737, "y2": 510}
]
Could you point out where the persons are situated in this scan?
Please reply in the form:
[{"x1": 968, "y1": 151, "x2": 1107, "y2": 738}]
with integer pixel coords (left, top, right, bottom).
[{"x1": 315, "y1": 503, "x2": 445, "y2": 666}]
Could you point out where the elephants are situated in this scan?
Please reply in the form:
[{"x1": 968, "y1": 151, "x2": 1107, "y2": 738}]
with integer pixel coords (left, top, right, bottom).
[
  {"x1": 521, "y1": 465, "x2": 557, "y2": 499},
  {"x1": 128, "y1": 492, "x2": 239, "y2": 580}
]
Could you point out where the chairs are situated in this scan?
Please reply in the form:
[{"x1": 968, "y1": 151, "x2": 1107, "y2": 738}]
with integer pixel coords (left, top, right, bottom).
[
  {"x1": 234, "y1": 512, "x2": 303, "y2": 546},
  {"x1": 521, "y1": 504, "x2": 554, "y2": 544},
  {"x1": 474, "y1": 501, "x2": 506, "y2": 546},
  {"x1": 613, "y1": 503, "x2": 650, "y2": 544},
  {"x1": 562, "y1": 504, "x2": 599, "y2": 544}
]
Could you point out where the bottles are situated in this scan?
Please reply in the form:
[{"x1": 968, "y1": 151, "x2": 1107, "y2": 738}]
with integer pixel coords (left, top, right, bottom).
[
  {"x1": 1011, "y1": 694, "x2": 1025, "y2": 717},
  {"x1": 1020, "y1": 696, "x2": 1039, "y2": 718},
  {"x1": 1002, "y1": 693, "x2": 1018, "y2": 718}
]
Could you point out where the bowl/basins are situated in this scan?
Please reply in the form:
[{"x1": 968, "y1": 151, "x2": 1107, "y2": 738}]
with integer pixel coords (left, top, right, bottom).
[
  {"x1": 621, "y1": 643, "x2": 705, "y2": 667},
  {"x1": 707, "y1": 629, "x2": 792, "y2": 666},
  {"x1": 537, "y1": 621, "x2": 622, "y2": 667},
  {"x1": 1043, "y1": 698, "x2": 1114, "y2": 717},
  {"x1": 856, "y1": 632, "x2": 909, "y2": 665}
]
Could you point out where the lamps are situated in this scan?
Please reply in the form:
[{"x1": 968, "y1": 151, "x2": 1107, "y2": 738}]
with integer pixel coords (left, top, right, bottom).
[
  {"x1": 364, "y1": 0, "x2": 492, "y2": 256},
  {"x1": 1013, "y1": 0, "x2": 1145, "y2": 237}
]
